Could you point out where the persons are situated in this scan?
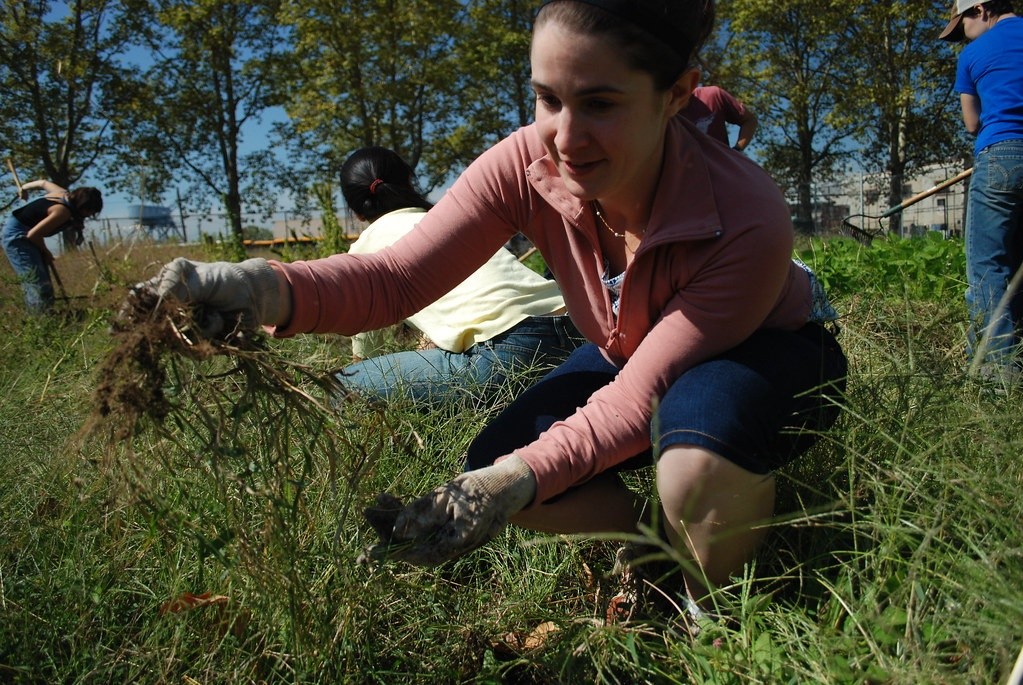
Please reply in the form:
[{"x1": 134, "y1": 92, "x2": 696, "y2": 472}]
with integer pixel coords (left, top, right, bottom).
[
  {"x1": 937, "y1": 0, "x2": 1023, "y2": 367},
  {"x1": 0, "y1": 179, "x2": 104, "y2": 310},
  {"x1": 312, "y1": 144, "x2": 586, "y2": 413},
  {"x1": 677, "y1": 86, "x2": 757, "y2": 155},
  {"x1": 108, "y1": 0, "x2": 847, "y2": 628}
]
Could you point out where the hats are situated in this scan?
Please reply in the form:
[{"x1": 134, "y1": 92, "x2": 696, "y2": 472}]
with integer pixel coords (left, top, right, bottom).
[{"x1": 939, "y1": 0, "x2": 990, "y2": 42}]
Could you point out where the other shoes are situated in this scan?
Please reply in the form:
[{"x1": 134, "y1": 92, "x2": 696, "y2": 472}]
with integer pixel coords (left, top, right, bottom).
[{"x1": 608, "y1": 547, "x2": 683, "y2": 621}]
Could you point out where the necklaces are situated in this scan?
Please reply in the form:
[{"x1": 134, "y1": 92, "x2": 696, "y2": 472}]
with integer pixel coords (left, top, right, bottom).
[{"x1": 593, "y1": 200, "x2": 648, "y2": 237}]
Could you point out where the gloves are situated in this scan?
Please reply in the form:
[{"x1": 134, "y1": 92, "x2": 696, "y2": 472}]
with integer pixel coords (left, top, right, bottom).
[
  {"x1": 356, "y1": 454, "x2": 537, "y2": 566},
  {"x1": 107, "y1": 258, "x2": 283, "y2": 358}
]
